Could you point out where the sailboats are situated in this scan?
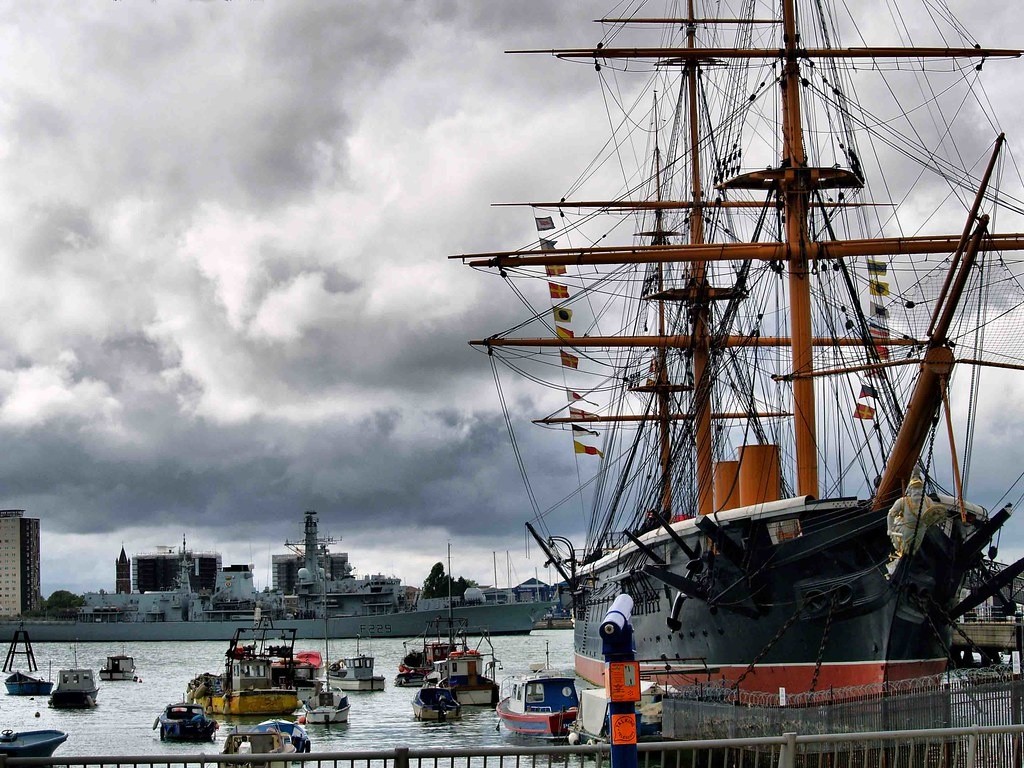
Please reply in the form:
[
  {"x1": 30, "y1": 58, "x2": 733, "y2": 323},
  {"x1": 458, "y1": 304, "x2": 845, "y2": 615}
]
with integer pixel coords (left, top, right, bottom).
[
  {"x1": 394, "y1": 543, "x2": 470, "y2": 688},
  {"x1": 447, "y1": 2, "x2": 1024, "y2": 767}
]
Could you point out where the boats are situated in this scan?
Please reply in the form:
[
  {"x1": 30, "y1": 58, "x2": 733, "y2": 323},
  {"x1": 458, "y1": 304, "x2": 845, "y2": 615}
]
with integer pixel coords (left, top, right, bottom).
[
  {"x1": 496, "y1": 639, "x2": 579, "y2": 741},
  {"x1": 0, "y1": 512, "x2": 561, "y2": 642},
  {"x1": 567, "y1": 681, "x2": 680, "y2": 761},
  {"x1": 422, "y1": 661, "x2": 450, "y2": 687},
  {"x1": 412, "y1": 688, "x2": 462, "y2": 720},
  {"x1": 435, "y1": 649, "x2": 500, "y2": 707},
  {"x1": 5, "y1": 669, "x2": 54, "y2": 697},
  {"x1": 0, "y1": 729, "x2": 68, "y2": 768},
  {"x1": 47, "y1": 669, "x2": 100, "y2": 709},
  {"x1": 153, "y1": 605, "x2": 386, "y2": 768},
  {"x1": 100, "y1": 656, "x2": 136, "y2": 680}
]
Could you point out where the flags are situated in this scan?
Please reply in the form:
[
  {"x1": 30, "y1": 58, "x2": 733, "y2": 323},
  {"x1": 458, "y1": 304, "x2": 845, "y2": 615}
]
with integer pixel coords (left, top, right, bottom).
[
  {"x1": 560, "y1": 350, "x2": 578, "y2": 369},
  {"x1": 853, "y1": 403, "x2": 875, "y2": 420},
  {"x1": 557, "y1": 325, "x2": 574, "y2": 337},
  {"x1": 865, "y1": 364, "x2": 885, "y2": 378},
  {"x1": 568, "y1": 389, "x2": 599, "y2": 406},
  {"x1": 870, "y1": 302, "x2": 890, "y2": 319},
  {"x1": 868, "y1": 260, "x2": 887, "y2": 276},
  {"x1": 549, "y1": 282, "x2": 569, "y2": 298},
  {"x1": 870, "y1": 279, "x2": 889, "y2": 296},
  {"x1": 575, "y1": 441, "x2": 604, "y2": 458},
  {"x1": 859, "y1": 385, "x2": 879, "y2": 399},
  {"x1": 539, "y1": 237, "x2": 557, "y2": 250},
  {"x1": 535, "y1": 216, "x2": 555, "y2": 231},
  {"x1": 554, "y1": 307, "x2": 572, "y2": 322},
  {"x1": 573, "y1": 424, "x2": 600, "y2": 437},
  {"x1": 570, "y1": 407, "x2": 600, "y2": 422},
  {"x1": 545, "y1": 265, "x2": 567, "y2": 276},
  {"x1": 870, "y1": 323, "x2": 889, "y2": 338},
  {"x1": 869, "y1": 346, "x2": 888, "y2": 360}
]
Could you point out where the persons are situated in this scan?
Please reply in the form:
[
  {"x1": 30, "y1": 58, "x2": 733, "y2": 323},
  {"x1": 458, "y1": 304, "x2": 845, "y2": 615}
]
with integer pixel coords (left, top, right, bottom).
[{"x1": 887, "y1": 480, "x2": 935, "y2": 553}]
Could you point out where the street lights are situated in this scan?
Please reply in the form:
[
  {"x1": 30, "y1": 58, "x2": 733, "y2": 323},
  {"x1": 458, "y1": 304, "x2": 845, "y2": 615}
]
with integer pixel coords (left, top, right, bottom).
[
  {"x1": 492, "y1": 550, "x2": 498, "y2": 605},
  {"x1": 43, "y1": 607, "x2": 49, "y2": 621}
]
